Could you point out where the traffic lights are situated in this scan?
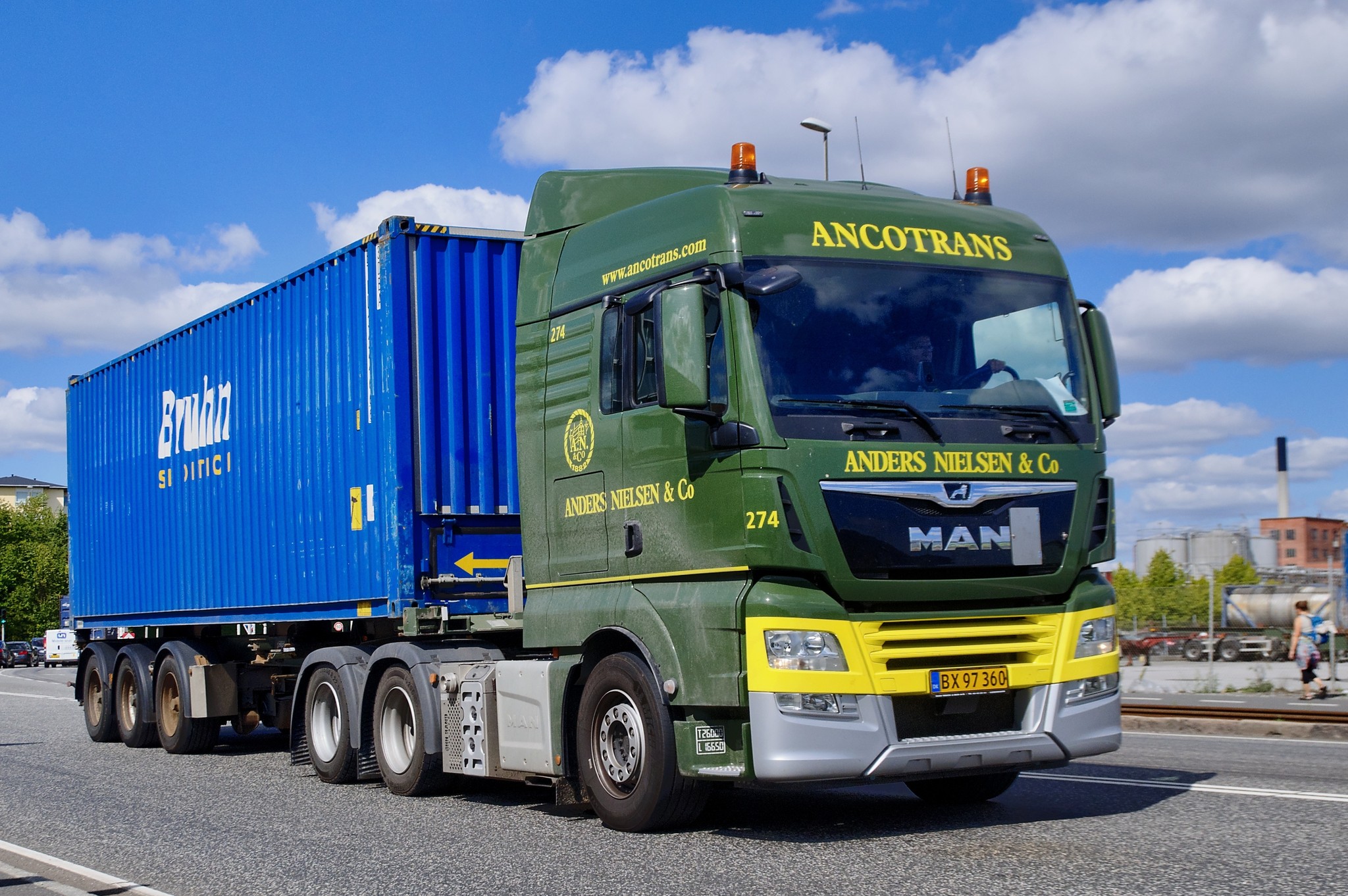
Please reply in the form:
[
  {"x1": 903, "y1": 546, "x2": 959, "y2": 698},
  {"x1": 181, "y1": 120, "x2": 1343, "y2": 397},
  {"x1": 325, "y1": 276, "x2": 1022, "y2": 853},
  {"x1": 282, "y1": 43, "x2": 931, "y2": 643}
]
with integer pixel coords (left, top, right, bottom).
[{"x1": 1, "y1": 610, "x2": 6, "y2": 624}]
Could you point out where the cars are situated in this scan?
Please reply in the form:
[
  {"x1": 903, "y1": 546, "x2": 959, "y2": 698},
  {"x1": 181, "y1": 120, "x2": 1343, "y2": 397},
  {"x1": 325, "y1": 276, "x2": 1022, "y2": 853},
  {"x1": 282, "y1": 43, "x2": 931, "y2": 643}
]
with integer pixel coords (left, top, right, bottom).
[
  {"x1": 30, "y1": 637, "x2": 44, "y2": 662},
  {"x1": 0, "y1": 641, "x2": 15, "y2": 669},
  {"x1": 5, "y1": 641, "x2": 39, "y2": 668}
]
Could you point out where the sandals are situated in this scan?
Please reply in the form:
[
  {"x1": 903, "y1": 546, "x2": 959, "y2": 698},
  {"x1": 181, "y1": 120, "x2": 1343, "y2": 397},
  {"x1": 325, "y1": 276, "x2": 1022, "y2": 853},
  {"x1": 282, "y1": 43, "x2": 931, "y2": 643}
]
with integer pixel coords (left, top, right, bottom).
[
  {"x1": 1299, "y1": 695, "x2": 1313, "y2": 700},
  {"x1": 1319, "y1": 686, "x2": 1327, "y2": 700}
]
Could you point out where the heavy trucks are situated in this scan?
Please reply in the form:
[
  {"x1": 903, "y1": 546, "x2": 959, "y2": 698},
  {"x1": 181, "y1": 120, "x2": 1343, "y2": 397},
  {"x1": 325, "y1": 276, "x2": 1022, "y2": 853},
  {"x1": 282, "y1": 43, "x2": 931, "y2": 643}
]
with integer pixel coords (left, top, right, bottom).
[{"x1": 64, "y1": 141, "x2": 1123, "y2": 835}]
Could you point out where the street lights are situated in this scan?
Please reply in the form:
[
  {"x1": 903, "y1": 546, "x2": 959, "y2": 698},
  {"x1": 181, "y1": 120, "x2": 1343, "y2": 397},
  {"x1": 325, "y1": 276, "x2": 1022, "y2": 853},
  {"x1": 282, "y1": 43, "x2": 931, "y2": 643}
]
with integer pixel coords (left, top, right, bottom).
[{"x1": 799, "y1": 117, "x2": 833, "y2": 181}]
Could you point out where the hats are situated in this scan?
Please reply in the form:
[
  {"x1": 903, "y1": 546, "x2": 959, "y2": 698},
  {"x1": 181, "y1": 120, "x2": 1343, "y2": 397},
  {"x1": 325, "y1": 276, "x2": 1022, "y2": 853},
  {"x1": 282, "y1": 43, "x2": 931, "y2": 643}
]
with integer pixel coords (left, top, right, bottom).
[{"x1": 1323, "y1": 619, "x2": 1338, "y2": 635}]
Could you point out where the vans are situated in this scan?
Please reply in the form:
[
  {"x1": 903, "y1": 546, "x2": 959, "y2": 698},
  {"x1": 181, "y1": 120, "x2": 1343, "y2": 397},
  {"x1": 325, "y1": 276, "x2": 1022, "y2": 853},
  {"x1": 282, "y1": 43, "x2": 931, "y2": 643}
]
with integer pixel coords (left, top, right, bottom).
[{"x1": 43, "y1": 630, "x2": 78, "y2": 668}]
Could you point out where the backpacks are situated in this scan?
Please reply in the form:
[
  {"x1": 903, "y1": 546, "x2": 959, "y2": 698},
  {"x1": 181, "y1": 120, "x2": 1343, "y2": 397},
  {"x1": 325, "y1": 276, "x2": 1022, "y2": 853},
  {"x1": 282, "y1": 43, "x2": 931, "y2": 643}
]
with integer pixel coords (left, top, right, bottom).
[{"x1": 1300, "y1": 614, "x2": 1329, "y2": 646}]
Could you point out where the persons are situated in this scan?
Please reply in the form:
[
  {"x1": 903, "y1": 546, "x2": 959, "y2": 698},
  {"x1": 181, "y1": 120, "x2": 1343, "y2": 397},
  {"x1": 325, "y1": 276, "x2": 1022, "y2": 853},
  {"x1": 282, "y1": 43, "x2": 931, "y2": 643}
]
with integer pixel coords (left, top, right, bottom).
[
  {"x1": 906, "y1": 335, "x2": 1006, "y2": 389},
  {"x1": 1288, "y1": 600, "x2": 1328, "y2": 700}
]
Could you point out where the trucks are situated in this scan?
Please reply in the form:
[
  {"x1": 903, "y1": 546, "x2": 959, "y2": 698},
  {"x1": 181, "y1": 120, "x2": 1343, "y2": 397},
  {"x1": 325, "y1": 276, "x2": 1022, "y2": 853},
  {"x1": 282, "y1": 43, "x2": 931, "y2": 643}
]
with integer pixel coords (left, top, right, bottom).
[
  {"x1": 1118, "y1": 623, "x2": 1290, "y2": 663},
  {"x1": 60, "y1": 597, "x2": 104, "y2": 650}
]
